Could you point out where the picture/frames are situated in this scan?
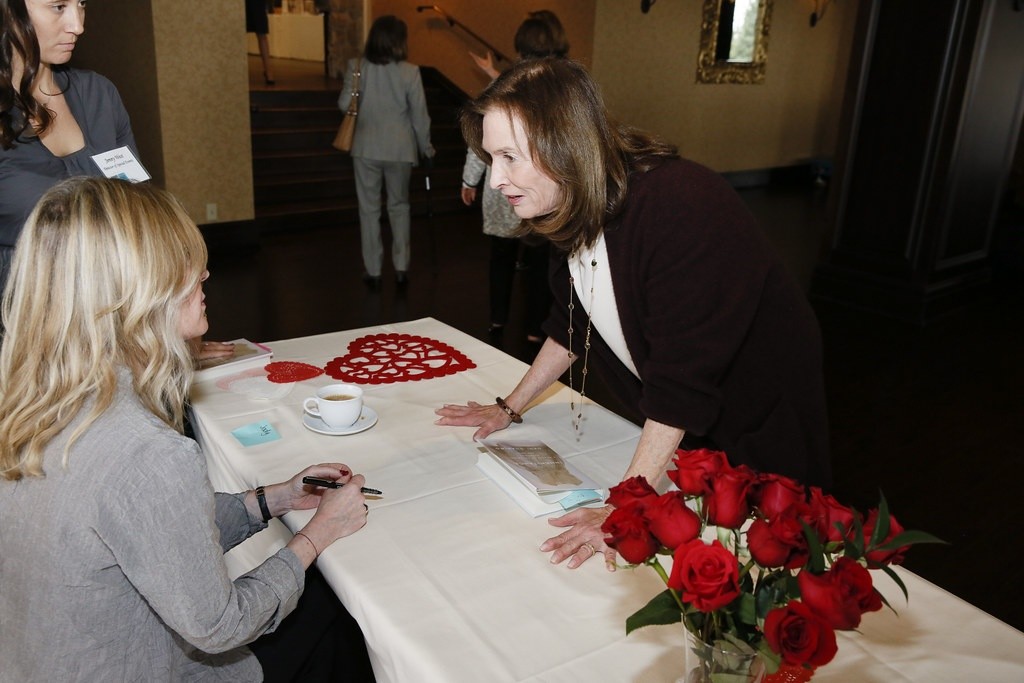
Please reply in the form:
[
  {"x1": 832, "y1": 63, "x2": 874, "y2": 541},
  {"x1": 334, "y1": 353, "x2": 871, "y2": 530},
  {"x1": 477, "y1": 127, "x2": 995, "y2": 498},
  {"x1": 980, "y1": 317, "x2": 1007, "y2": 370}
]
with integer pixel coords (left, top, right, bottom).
[{"x1": 695, "y1": 0, "x2": 775, "y2": 86}]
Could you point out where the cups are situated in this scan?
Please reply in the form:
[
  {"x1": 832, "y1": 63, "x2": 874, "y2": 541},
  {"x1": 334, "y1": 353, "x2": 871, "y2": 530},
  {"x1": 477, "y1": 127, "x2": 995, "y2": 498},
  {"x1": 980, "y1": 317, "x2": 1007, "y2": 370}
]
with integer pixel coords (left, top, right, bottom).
[{"x1": 304, "y1": 383, "x2": 364, "y2": 430}]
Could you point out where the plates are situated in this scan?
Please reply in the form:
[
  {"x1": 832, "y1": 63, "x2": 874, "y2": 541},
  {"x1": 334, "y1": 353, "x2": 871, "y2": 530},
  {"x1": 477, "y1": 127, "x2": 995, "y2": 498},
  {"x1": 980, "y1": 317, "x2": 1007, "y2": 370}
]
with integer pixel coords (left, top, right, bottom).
[{"x1": 302, "y1": 405, "x2": 378, "y2": 436}]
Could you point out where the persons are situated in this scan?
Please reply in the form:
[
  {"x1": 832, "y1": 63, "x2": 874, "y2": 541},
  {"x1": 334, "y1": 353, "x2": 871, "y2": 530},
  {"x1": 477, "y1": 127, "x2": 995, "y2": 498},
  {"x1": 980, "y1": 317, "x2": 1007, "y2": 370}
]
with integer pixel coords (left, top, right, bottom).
[
  {"x1": 435, "y1": 57, "x2": 835, "y2": 572},
  {"x1": 246, "y1": 0, "x2": 282, "y2": 85},
  {"x1": 459, "y1": 9, "x2": 570, "y2": 341},
  {"x1": 0, "y1": 173, "x2": 367, "y2": 683},
  {"x1": 0, "y1": 0, "x2": 234, "y2": 361},
  {"x1": 336, "y1": 14, "x2": 435, "y2": 288}
]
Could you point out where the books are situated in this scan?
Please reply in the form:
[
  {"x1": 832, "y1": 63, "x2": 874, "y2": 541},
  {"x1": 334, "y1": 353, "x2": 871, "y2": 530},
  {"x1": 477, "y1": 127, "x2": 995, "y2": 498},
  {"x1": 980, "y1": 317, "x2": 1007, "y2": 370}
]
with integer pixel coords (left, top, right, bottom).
[{"x1": 191, "y1": 338, "x2": 273, "y2": 384}]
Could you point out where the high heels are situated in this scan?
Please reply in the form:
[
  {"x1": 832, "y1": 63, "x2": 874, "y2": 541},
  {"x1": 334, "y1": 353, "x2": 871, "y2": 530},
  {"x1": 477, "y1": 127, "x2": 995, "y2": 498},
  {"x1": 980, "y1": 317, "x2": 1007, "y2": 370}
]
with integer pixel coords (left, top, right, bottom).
[{"x1": 264, "y1": 71, "x2": 275, "y2": 87}]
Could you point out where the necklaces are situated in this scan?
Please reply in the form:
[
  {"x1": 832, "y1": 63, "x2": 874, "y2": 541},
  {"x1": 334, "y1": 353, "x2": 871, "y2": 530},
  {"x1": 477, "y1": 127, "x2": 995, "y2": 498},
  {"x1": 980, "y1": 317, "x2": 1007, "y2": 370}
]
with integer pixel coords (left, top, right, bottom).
[{"x1": 567, "y1": 237, "x2": 597, "y2": 431}]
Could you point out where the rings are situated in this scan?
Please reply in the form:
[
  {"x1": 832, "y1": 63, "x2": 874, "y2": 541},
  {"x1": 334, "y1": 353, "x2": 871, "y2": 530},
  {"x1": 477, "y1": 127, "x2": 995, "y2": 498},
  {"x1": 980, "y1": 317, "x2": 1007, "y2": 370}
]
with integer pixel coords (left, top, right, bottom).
[
  {"x1": 581, "y1": 543, "x2": 595, "y2": 555},
  {"x1": 204, "y1": 341, "x2": 208, "y2": 345},
  {"x1": 364, "y1": 504, "x2": 369, "y2": 514}
]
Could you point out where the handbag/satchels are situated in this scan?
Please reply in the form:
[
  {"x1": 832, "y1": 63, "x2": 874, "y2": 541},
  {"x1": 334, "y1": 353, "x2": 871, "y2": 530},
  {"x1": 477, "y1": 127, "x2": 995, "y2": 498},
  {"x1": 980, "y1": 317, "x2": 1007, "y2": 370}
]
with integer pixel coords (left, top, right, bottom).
[{"x1": 332, "y1": 55, "x2": 362, "y2": 152}]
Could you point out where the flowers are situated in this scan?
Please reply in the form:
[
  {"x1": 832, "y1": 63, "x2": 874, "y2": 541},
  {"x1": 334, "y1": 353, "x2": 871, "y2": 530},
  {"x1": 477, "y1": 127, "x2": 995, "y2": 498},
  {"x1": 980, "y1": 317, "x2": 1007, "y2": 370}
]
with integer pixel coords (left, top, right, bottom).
[{"x1": 600, "y1": 445, "x2": 947, "y2": 683}]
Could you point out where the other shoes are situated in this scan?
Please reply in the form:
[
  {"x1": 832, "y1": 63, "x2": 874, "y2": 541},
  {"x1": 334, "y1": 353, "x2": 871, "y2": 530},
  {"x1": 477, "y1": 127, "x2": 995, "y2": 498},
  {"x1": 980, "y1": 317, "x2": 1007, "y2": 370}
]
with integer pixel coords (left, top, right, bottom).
[
  {"x1": 486, "y1": 324, "x2": 504, "y2": 345},
  {"x1": 360, "y1": 271, "x2": 385, "y2": 292},
  {"x1": 526, "y1": 331, "x2": 550, "y2": 350},
  {"x1": 393, "y1": 271, "x2": 408, "y2": 291}
]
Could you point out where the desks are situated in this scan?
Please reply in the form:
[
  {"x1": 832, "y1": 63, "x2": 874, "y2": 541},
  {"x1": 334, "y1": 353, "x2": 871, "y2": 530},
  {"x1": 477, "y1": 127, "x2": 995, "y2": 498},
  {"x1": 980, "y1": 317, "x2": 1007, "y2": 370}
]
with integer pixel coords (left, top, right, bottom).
[{"x1": 153, "y1": 317, "x2": 1024, "y2": 683}]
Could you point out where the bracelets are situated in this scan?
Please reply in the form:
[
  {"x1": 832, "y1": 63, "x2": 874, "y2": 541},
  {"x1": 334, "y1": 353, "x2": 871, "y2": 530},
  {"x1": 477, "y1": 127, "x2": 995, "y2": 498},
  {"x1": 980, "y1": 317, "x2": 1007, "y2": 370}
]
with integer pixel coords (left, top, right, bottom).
[
  {"x1": 496, "y1": 397, "x2": 523, "y2": 423},
  {"x1": 256, "y1": 486, "x2": 272, "y2": 521},
  {"x1": 297, "y1": 532, "x2": 318, "y2": 556}
]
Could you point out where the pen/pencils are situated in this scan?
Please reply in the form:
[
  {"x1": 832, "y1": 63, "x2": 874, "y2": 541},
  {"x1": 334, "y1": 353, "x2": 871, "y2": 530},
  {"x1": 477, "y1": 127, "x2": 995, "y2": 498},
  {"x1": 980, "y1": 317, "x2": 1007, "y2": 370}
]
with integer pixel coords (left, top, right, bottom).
[{"x1": 302, "y1": 476, "x2": 382, "y2": 495}]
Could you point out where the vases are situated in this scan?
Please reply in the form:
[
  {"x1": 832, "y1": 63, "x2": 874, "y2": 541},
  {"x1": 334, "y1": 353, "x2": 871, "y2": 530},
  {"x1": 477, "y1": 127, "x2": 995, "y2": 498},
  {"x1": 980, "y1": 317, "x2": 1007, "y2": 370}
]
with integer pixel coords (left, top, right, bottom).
[{"x1": 678, "y1": 613, "x2": 762, "y2": 683}]
